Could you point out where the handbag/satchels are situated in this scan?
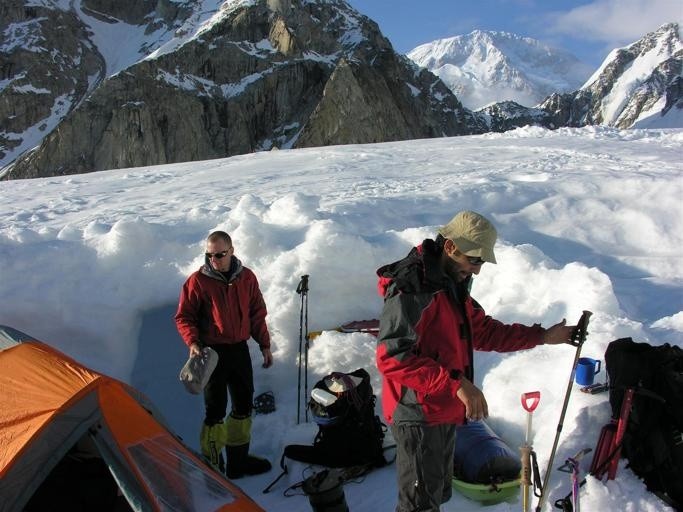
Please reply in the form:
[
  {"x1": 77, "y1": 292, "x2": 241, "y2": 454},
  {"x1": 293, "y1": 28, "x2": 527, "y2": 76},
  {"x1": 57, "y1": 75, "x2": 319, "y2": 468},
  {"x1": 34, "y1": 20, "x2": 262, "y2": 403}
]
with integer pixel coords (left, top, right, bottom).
[
  {"x1": 605, "y1": 337, "x2": 682, "y2": 511},
  {"x1": 307, "y1": 368, "x2": 387, "y2": 468}
]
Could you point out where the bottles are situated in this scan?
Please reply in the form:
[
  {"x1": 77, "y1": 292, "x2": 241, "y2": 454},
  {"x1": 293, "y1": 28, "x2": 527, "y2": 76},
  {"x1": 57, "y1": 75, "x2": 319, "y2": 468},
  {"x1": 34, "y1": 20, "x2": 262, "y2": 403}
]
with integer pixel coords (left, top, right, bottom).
[{"x1": 309, "y1": 387, "x2": 338, "y2": 408}]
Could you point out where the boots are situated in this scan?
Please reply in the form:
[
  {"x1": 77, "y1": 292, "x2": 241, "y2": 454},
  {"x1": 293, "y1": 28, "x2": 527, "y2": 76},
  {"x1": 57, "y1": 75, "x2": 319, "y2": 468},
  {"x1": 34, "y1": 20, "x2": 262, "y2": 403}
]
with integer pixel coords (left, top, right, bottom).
[{"x1": 226, "y1": 443, "x2": 271, "y2": 479}]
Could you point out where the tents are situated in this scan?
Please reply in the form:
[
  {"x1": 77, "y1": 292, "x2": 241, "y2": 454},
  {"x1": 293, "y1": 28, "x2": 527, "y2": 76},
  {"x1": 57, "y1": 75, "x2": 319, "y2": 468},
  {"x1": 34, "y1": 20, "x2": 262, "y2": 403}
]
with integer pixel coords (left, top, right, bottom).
[{"x1": 0, "y1": 323, "x2": 269, "y2": 512}]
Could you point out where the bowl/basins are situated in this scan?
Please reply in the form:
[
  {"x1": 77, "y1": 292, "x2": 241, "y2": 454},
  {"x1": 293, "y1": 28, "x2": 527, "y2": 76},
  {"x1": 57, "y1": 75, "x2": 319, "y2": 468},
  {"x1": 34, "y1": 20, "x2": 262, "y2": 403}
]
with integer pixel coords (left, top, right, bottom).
[{"x1": 452, "y1": 474, "x2": 523, "y2": 501}]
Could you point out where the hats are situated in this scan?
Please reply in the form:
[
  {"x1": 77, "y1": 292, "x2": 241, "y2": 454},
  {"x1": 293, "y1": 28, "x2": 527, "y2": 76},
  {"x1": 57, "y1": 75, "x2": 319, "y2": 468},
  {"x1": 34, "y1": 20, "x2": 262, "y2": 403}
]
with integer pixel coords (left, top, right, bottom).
[{"x1": 437, "y1": 211, "x2": 497, "y2": 263}]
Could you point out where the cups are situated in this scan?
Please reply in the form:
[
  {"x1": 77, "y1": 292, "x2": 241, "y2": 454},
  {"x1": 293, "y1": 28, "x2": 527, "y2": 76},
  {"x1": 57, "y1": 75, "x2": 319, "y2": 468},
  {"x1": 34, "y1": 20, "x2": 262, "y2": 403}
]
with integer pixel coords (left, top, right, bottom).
[{"x1": 575, "y1": 358, "x2": 601, "y2": 385}]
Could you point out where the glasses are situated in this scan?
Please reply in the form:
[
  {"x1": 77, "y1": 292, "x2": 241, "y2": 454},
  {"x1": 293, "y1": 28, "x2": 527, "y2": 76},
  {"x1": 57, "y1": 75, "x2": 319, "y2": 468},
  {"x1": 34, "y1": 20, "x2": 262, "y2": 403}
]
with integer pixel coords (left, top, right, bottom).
[
  {"x1": 455, "y1": 248, "x2": 483, "y2": 265},
  {"x1": 205, "y1": 247, "x2": 230, "y2": 259}
]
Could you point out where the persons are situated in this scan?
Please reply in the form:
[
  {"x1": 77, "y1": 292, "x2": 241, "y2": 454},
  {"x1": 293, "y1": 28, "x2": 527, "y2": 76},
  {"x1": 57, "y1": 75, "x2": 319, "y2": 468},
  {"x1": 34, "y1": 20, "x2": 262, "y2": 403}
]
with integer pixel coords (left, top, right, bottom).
[
  {"x1": 373, "y1": 208, "x2": 589, "y2": 511},
  {"x1": 171, "y1": 230, "x2": 275, "y2": 501}
]
